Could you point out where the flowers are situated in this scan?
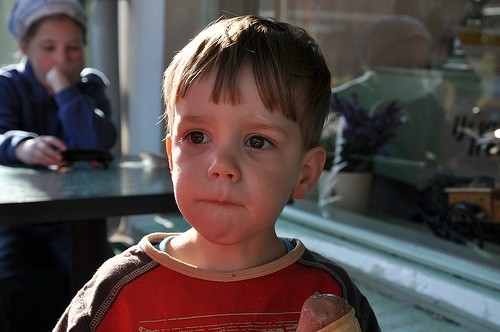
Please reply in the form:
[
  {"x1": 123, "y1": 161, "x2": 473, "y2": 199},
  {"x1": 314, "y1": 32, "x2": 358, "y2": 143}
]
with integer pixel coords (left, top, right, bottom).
[{"x1": 318, "y1": 90, "x2": 404, "y2": 176}]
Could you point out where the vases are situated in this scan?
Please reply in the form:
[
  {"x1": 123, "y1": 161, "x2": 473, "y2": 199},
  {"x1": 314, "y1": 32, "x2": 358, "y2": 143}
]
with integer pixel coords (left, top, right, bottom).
[{"x1": 315, "y1": 169, "x2": 372, "y2": 216}]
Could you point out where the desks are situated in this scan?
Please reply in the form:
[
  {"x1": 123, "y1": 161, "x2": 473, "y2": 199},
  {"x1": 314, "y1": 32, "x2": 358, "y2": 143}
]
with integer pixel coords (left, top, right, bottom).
[{"x1": 0, "y1": 156, "x2": 184, "y2": 296}]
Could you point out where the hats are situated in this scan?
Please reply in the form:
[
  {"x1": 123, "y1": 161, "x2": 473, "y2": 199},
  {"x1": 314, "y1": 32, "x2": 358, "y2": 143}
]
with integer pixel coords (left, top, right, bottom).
[
  {"x1": 8, "y1": 0, "x2": 89, "y2": 43},
  {"x1": 359, "y1": 16, "x2": 433, "y2": 70}
]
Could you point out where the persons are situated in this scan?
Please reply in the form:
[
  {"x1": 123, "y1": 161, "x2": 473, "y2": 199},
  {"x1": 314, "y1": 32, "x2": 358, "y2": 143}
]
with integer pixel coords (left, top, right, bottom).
[
  {"x1": 0, "y1": 0, "x2": 115, "y2": 332},
  {"x1": 49, "y1": 15, "x2": 380, "y2": 332},
  {"x1": 322, "y1": 16, "x2": 500, "y2": 207}
]
